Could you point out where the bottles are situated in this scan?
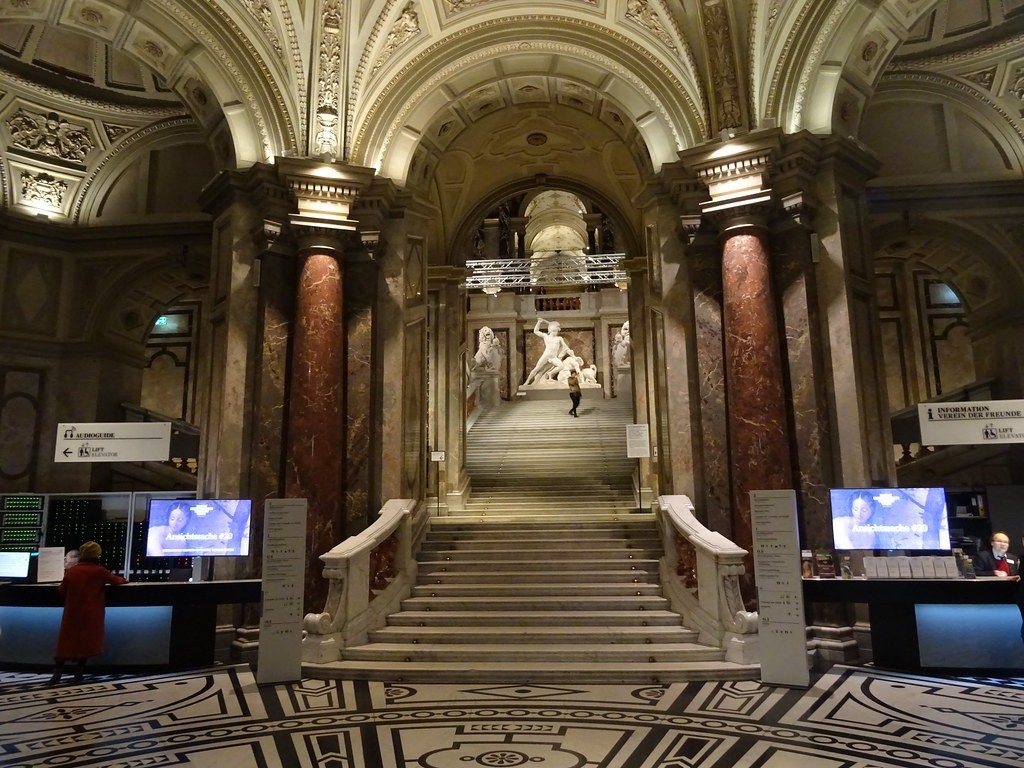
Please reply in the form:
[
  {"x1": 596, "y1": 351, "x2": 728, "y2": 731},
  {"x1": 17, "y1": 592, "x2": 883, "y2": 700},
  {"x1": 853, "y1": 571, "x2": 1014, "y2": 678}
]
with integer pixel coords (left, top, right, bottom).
[
  {"x1": 967, "y1": 559, "x2": 973, "y2": 572},
  {"x1": 963, "y1": 555, "x2": 968, "y2": 575},
  {"x1": 841, "y1": 556, "x2": 852, "y2": 579}
]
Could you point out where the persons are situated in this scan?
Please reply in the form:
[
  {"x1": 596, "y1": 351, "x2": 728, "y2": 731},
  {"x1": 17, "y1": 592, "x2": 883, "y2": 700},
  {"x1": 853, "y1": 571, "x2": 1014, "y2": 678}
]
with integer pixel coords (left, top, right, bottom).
[
  {"x1": 569, "y1": 371, "x2": 582, "y2": 417},
  {"x1": 523, "y1": 317, "x2": 584, "y2": 385},
  {"x1": 971, "y1": 532, "x2": 1018, "y2": 577},
  {"x1": 47, "y1": 543, "x2": 126, "y2": 685},
  {"x1": 803, "y1": 562, "x2": 812, "y2": 577},
  {"x1": 65, "y1": 550, "x2": 81, "y2": 571}
]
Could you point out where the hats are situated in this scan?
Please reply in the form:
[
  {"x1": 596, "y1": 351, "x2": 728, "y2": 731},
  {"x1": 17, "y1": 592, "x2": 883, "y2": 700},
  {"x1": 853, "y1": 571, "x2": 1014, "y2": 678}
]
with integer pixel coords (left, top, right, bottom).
[{"x1": 79, "y1": 541, "x2": 102, "y2": 559}]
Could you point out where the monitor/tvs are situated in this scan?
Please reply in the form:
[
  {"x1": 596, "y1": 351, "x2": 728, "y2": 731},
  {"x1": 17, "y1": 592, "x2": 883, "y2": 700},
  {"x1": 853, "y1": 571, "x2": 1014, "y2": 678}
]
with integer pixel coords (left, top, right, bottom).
[
  {"x1": 0, "y1": 552, "x2": 31, "y2": 578},
  {"x1": 828, "y1": 487, "x2": 952, "y2": 557},
  {"x1": 144, "y1": 497, "x2": 253, "y2": 556}
]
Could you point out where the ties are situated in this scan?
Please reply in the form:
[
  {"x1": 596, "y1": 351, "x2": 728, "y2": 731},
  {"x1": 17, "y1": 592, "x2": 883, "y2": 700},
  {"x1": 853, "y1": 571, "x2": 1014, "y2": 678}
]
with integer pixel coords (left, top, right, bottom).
[{"x1": 997, "y1": 555, "x2": 1005, "y2": 561}]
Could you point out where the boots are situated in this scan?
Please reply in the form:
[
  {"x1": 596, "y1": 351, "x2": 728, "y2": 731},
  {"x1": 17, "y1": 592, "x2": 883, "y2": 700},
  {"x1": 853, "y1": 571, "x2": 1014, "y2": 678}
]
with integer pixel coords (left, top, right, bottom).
[
  {"x1": 47, "y1": 664, "x2": 65, "y2": 685},
  {"x1": 73, "y1": 661, "x2": 89, "y2": 682}
]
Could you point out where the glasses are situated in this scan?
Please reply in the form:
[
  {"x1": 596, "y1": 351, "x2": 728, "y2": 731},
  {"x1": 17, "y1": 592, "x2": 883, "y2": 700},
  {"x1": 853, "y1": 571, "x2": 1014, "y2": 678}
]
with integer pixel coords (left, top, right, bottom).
[{"x1": 992, "y1": 539, "x2": 1009, "y2": 544}]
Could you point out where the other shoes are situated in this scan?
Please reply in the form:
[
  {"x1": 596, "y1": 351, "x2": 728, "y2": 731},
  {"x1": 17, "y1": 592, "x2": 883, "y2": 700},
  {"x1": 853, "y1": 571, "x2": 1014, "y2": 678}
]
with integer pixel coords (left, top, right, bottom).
[
  {"x1": 569, "y1": 410, "x2": 574, "y2": 415},
  {"x1": 574, "y1": 414, "x2": 579, "y2": 417}
]
